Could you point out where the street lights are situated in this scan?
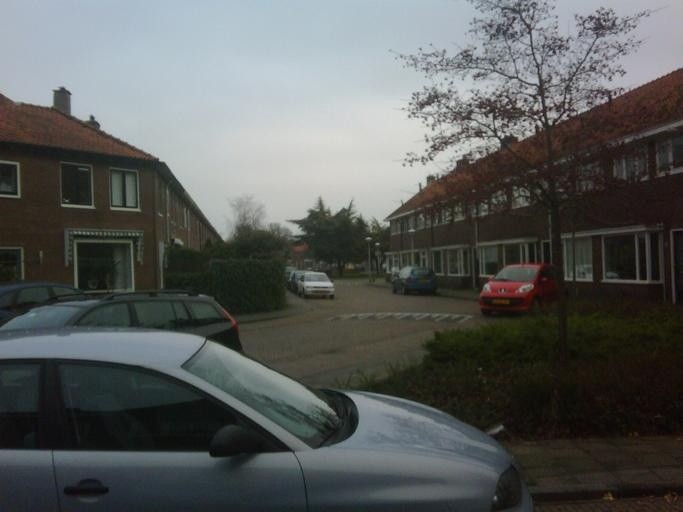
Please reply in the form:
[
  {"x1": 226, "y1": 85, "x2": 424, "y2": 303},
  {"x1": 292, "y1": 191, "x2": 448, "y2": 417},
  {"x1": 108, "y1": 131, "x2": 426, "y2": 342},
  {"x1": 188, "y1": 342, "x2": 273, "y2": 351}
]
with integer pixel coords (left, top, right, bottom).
[
  {"x1": 375, "y1": 243, "x2": 381, "y2": 273},
  {"x1": 365, "y1": 237, "x2": 373, "y2": 283}
]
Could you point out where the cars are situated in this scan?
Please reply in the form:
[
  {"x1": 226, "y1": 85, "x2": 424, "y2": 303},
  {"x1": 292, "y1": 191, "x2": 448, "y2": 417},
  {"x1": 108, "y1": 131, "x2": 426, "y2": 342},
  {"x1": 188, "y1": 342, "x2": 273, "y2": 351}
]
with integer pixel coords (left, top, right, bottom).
[
  {"x1": 0, "y1": 328, "x2": 534, "y2": 511},
  {"x1": 479, "y1": 263, "x2": 558, "y2": 315},
  {"x1": 288, "y1": 271, "x2": 335, "y2": 298},
  {"x1": 393, "y1": 267, "x2": 436, "y2": 294}
]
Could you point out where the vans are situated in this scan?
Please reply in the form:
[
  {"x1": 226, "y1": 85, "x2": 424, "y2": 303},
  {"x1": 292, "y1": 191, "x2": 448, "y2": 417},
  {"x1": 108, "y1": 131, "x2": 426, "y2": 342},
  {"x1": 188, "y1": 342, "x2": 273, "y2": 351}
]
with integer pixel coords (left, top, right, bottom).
[{"x1": 0, "y1": 284, "x2": 244, "y2": 353}]
